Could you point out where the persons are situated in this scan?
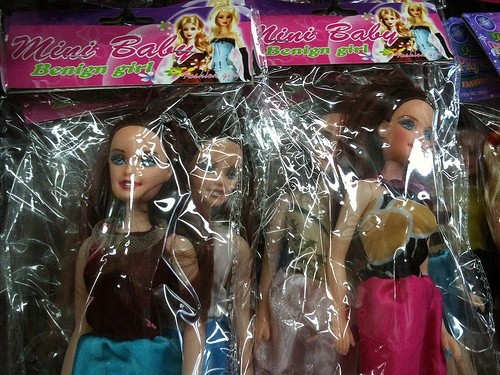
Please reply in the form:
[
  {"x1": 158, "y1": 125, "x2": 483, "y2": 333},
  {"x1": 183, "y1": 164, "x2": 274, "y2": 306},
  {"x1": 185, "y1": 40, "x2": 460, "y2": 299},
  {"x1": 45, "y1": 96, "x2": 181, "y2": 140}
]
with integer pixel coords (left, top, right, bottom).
[
  {"x1": 326, "y1": 78, "x2": 485, "y2": 374},
  {"x1": 178, "y1": 129, "x2": 254, "y2": 375},
  {"x1": 58, "y1": 113, "x2": 214, "y2": 375},
  {"x1": 253, "y1": 80, "x2": 348, "y2": 375}
]
